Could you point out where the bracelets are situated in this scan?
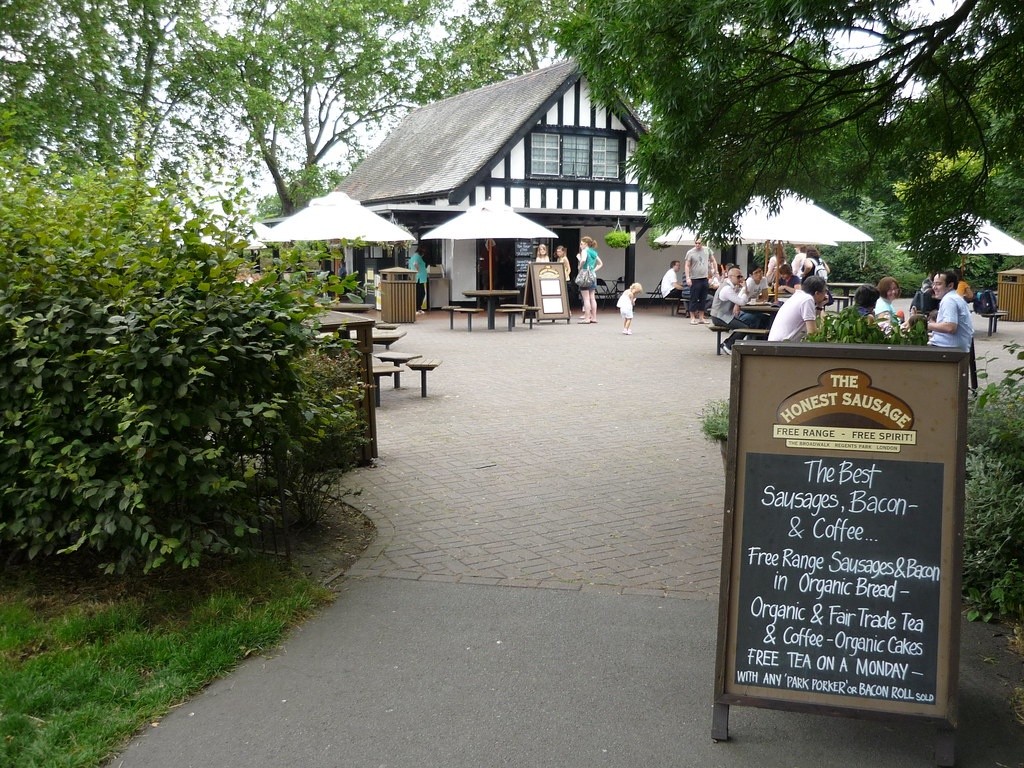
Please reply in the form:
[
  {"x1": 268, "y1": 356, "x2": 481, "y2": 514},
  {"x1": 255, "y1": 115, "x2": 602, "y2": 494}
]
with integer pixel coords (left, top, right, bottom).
[
  {"x1": 815, "y1": 307, "x2": 822, "y2": 311},
  {"x1": 714, "y1": 272, "x2": 719, "y2": 274}
]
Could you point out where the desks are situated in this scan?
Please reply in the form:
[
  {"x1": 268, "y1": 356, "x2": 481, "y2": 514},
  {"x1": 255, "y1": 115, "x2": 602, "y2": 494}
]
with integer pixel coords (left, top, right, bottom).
[
  {"x1": 307, "y1": 310, "x2": 379, "y2": 461},
  {"x1": 462, "y1": 290, "x2": 520, "y2": 331},
  {"x1": 706, "y1": 305, "x2": 781, "y2": 311},
  {"x1": 826, "y1": 282, "x2": 870, "y2": 310}
]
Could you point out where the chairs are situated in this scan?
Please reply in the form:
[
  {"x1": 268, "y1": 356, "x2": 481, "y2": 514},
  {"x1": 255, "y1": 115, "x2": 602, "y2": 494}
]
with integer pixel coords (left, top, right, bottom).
[
  {"x1": 643, "y1": 280, "x2": 662, "y2": 309},
  {"x1": 592, "y1": 277, "x2": 630, "y2": 310}
]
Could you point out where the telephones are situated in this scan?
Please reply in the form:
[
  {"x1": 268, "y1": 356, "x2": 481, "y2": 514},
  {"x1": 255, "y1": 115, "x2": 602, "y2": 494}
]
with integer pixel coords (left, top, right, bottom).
[{"x1": 515, "y1": 242, "x2": 533, "y2": 256}]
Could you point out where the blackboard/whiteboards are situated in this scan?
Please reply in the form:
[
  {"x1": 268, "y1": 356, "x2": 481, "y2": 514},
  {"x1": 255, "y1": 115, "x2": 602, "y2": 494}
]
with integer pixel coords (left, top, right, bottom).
[
  {"x1": 515, "y1": 257, "x2": 534, "y2": 273},
  {"x1": 515, "y1": 273, "x2": 528, "y2": 289},
  {"x1": 723, "y1": 344, "x2": 970, "y2": 728}
]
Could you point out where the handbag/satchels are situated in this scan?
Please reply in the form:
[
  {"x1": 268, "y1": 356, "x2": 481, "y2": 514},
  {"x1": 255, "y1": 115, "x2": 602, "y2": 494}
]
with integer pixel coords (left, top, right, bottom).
[{"x1": 575, "y1": 255, "x2": 595, "y2": 288}]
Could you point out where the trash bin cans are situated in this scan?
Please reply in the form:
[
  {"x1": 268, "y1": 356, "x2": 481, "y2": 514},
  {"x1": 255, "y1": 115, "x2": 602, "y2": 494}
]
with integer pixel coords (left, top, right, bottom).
[
  {"x1": 378, "y1": 267, "x2": 418, "y2": 323},
  {"x1": 997, "y1": 268, "x2": 1024, "y2": 322},
  {"x1": 300, "y1": 311, "x2": 379, "y2": 469}
]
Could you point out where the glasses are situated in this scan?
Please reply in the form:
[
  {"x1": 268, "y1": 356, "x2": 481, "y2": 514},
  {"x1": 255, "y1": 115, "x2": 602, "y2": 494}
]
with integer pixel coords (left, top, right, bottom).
[
  {"x1": 730, "y1": 274, "x2": 743, "y2": 279},
  {"x1": 753, "y1": 270, "x2": 761, "y2": 274},
  {"x1": 695, "y1": 242, "x2": 700, "y2": 244},
  {"x1": 887, "y1": 289, "x2": 899, "y2": 292}
]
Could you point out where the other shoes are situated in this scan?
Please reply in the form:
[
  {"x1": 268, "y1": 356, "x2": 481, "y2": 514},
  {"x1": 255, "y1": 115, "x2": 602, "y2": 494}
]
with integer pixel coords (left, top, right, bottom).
[
  {"x1": 720, "y1": 342, "x2": 732, "y2": 356},
  {"x1": 416, "y1": 310, "x2": 425, "y2": 315},
  {"x1": 579, "y1": 315, "x2": 585, "y2": 319},
  {"x1": 690, "y1": 320, "x2": 697, "y2": 325},
  {"x1": 590, "y1": 321, "x2": 597, "y2": 323},
  {"x1": 577, "y1": 320, "x2": 590, "y2": 324},
  {"x1": 698, "y1": 319, "x2": 709, "y2": 324},
  {"x1": 623, "y1": 329, "x2": 632, "y2": 335}
]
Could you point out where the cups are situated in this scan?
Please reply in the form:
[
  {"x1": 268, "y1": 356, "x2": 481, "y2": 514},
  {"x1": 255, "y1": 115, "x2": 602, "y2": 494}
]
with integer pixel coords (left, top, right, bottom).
[{"x1": 761, "y1": 288, "x2": 768, "y2": 302}]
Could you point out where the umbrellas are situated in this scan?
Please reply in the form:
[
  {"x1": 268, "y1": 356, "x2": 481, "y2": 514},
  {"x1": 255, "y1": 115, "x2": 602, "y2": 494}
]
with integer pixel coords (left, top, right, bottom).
[
  {"x1": 894, "y1": 214, "x2": 1024, "y2": 276},
  {"x1": 654, "y1": 189, "x2": 874, "y2": 304},
  {"x1": 259, "y1": 190, "x2": 417, "y2": 294},
  {"x1": 421, "y1": 197, "x2": 559, "y2": 291}
]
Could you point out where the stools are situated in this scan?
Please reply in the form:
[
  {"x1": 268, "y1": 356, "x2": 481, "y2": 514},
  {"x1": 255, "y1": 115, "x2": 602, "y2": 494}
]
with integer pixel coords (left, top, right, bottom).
[
  {"x1": 371, "y1": 323, "x2": 443, "y2": 407},
  {"x1": 981, "y1": 310, "x2": 1008, "y2": 336},
  {"x1": 669, "y1": 294, "x2": 856, "y2": 355},
  {"x1": 440, "y1": 304, "x2": 542, "y2": 332}
]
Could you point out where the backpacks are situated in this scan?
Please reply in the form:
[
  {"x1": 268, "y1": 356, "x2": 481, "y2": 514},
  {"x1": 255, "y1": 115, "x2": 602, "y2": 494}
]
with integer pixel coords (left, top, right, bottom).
[
  {"x1": 974, "y1": 289, "x2": 998, "y2": 314},
  {"x1": 808, "y1": 257, "x2": 828, "y2": 283}
]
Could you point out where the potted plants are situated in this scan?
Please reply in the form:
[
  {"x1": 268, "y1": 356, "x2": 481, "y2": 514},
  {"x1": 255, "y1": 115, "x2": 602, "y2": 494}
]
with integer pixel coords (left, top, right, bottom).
[
  {"x1": 605, "y1": 231, "x2": 631, "y2": 248},
  {"x1": 699, "y1": 395, "x2": 730, "y2": 466},
  {"x1": 647, "y1": 227, "x2": 671, "y2": 250}
]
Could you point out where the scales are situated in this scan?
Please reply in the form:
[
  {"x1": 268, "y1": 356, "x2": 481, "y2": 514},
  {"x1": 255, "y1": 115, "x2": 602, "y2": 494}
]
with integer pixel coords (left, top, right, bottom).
[{"x1": 427, "y1": 264, "x2": 445, "y2": 278}]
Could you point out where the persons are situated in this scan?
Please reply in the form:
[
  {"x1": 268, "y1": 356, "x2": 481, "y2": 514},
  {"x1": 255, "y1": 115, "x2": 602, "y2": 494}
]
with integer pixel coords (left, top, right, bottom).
[
  {"x1": 408, "y1": 245, "x2": 427, "y2": 315},
  {"x1": 616, "y1": 282, "x2": 643, "y2": 335},
  {"x1": 853, "y1": 284, "x2": 881, "y2": 325},
  {"x1": 576, "y1": 237, "x2": 603, "y2": 324},
  {"x1": 685, "y1": 236, "x2": 721, "y2": 325},
  {"x1": 536, "y1": 244, "x2": 549, "y2": 262},
  {"x1": 710, "y1": 244, "x2": 831, "y2": 356},
  {"x1": 553, "y1": 245, "x2": 571, "y2": 285},
  {"x1": 875, "y1": 270, "x2": 974, "y2": 353},
  {"x1": 661, "y1": 261, "x2": 690, "y2": 318}
]
